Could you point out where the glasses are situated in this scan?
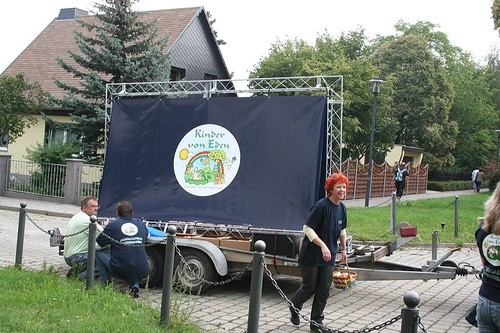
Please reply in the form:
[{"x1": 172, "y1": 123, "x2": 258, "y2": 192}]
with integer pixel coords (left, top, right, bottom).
[{"x1": 87, "y1": 205, "x2": 99, "y2": 210}]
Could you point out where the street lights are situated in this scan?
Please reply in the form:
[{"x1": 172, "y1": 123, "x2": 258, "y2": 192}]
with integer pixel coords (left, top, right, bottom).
[{"x1": 365, "y1": 79, "x2": 386, "y2": 207}]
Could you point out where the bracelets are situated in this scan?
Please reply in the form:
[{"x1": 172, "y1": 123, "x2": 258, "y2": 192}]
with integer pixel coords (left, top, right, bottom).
[{"x1": 341, "y1": 248, "x2": 346, "y2": 250}]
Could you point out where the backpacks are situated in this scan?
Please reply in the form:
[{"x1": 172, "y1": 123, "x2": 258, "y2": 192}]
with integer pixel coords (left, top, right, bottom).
[{"x1": 394, "y1": 168, "x2": 406, "y2": 183}]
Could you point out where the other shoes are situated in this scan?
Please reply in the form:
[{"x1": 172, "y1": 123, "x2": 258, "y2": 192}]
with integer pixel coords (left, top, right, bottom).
[
  {"x1": 288, "y1": 303, "x2": 300, "y2": 325},
  {"x1": 129, "y1": 288, "x2": 138, "y2": 298},
  {"x1": 310, "y1": 326, "x2": 333, "y2": 333}
]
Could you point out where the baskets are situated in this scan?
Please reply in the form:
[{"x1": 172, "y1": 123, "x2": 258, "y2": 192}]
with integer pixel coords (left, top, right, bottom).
[{"x1": 332, "y1": 260, "x2": 357, "y2": 289}]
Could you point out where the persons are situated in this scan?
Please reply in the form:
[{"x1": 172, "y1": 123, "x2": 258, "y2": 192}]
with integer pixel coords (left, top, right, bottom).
[
  {"x1": 474, "y1": 180, "x2": 500, "y2": 333},
  {"x1": 288, "y1": 172, "x2": 349, "y2": 333},
  {"x1": 391, "y1": 162, "x2": 409, "y2": 201},
  {"x1": 475, "y1": 169, "x2": 484, "y2": 192},
  {"x1": 94, "y1": 200, "x2": 150, "y2": 295},
  {"x1": 471, "y1": 167, "x2": 480, "y2": 192},
  {"x1": 63, "y1": 196, "x2": 105, "y2": 282}
]
